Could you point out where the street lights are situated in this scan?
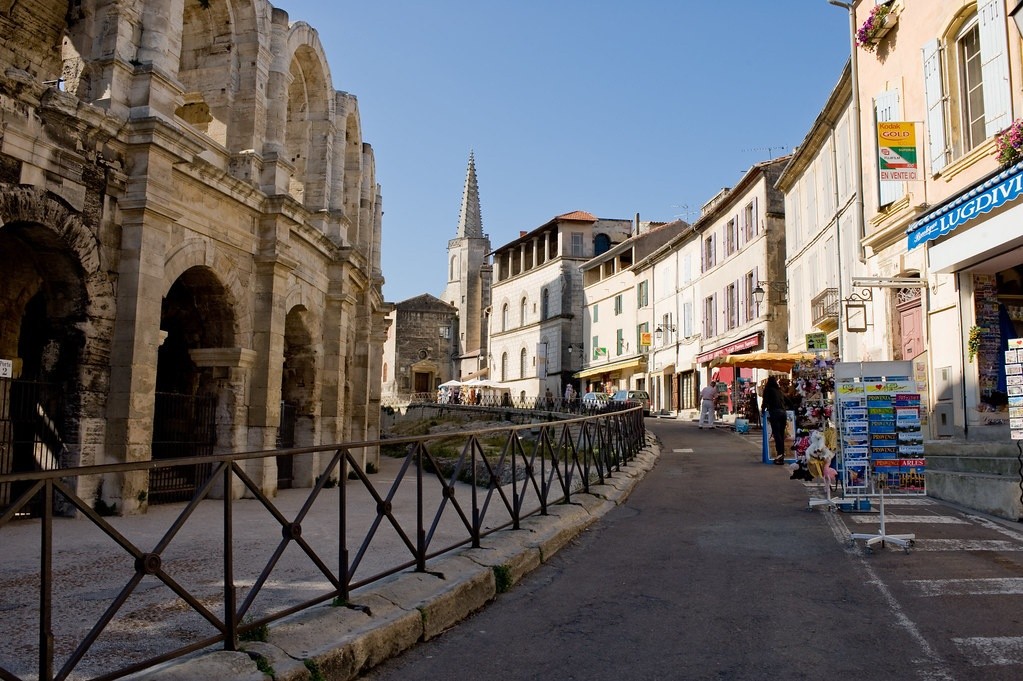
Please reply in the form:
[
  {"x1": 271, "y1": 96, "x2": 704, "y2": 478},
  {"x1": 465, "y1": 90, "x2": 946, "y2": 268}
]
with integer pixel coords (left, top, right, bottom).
[
  {"x1": 751, "y1": 280, "x2": 789, "y2": 306},
  {"x1": 479, "y1": 351, "x2": 492, "y2": 360},
  {"x1": 655, "y1": 324, "x2": 677, "y2": 339},
  {"x1": 568, "y1": 342, "x2": 584, "y2": 353}
]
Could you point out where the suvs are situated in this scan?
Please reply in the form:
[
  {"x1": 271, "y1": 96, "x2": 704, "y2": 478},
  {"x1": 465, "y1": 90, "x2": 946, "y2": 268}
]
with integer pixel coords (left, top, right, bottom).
[{"x1": 608, "y1": 390, "x2": 650, "y2": 417}]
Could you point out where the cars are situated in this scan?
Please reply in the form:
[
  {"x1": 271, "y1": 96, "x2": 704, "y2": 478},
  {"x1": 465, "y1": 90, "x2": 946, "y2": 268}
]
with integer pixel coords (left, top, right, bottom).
[{"x1": 581, "y1": 392, "x2": 614, "y2": 412}]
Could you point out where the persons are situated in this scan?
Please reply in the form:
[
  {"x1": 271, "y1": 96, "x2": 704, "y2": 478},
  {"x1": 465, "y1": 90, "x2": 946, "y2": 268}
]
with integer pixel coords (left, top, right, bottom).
[
  {"x1": 543, "y1": 388, "x2": 554, "y2": 412},
  {"x1": 697, "y1": 381, "x2": 717, "y2": 430},
  {"x1": 760, "y1": 377, "x2": 786, "y2": 467}
]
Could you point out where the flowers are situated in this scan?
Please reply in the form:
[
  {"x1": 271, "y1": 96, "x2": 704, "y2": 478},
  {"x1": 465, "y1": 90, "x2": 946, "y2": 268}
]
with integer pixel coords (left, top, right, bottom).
[
  {"x1": 968, "y1": 326, "x2": 982, "y2": 364},
  {"x1": 853, "y1": 4, "x2": 898, "y2": 53},
  {"x1": 992, "y1": 117, "x2": 1023, "y2": 170}
]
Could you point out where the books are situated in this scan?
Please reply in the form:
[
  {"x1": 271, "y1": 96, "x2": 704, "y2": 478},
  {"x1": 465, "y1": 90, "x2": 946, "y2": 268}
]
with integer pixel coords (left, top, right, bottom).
[{"x1": 836, "y1": 391, "x2": 924, "y2": 495}]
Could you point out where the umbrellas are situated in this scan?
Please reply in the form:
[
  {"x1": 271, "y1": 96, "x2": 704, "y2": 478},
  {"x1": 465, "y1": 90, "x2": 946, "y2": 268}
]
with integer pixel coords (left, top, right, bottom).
[{"x1": 438, "y1": 378, "x2": 509, "y2": 408}]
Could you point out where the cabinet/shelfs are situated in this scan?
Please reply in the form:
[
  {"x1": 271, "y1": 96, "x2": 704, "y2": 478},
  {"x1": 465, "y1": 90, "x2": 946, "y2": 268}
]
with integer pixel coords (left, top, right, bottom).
[{"x1": 833, "y1": 359, "x2": 928, "y2": 497}]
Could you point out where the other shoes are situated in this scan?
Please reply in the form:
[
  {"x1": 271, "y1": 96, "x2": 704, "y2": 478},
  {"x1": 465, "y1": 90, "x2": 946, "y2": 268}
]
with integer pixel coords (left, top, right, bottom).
[
  {"x1": 709, "y1": 427, "x2": 715, "y2": 429},
  {"x1": 775, "y1": 459, "x2": 784, "y2": 465},
  {"x1": 774, "y1": 453, "x2": 786, "y2": 461},
  {"x1": 699, "y1": 427, "x2": 703, "y2": 429}
]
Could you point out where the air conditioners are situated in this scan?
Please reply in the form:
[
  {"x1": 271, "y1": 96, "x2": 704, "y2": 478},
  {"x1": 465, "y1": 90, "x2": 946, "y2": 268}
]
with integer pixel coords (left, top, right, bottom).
[{"x1": 751, "y1": 280, "x2": 789, "y2": 306}]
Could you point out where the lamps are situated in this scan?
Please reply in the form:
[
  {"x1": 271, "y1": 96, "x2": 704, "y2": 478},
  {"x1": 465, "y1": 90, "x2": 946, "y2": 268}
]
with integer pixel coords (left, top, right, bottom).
[
  {"x1": 619, "y1": 337, "x2": 628, "y2": 351},
  {"x1": 567, "y1": 342, "x2": 584, "y2": 358},
  {"x1": 655, "y1": 323, "x2": 678, "y2": 338},
  {"x1": 480, "y1": 355, "x2": 491, "y2": 361}
]
